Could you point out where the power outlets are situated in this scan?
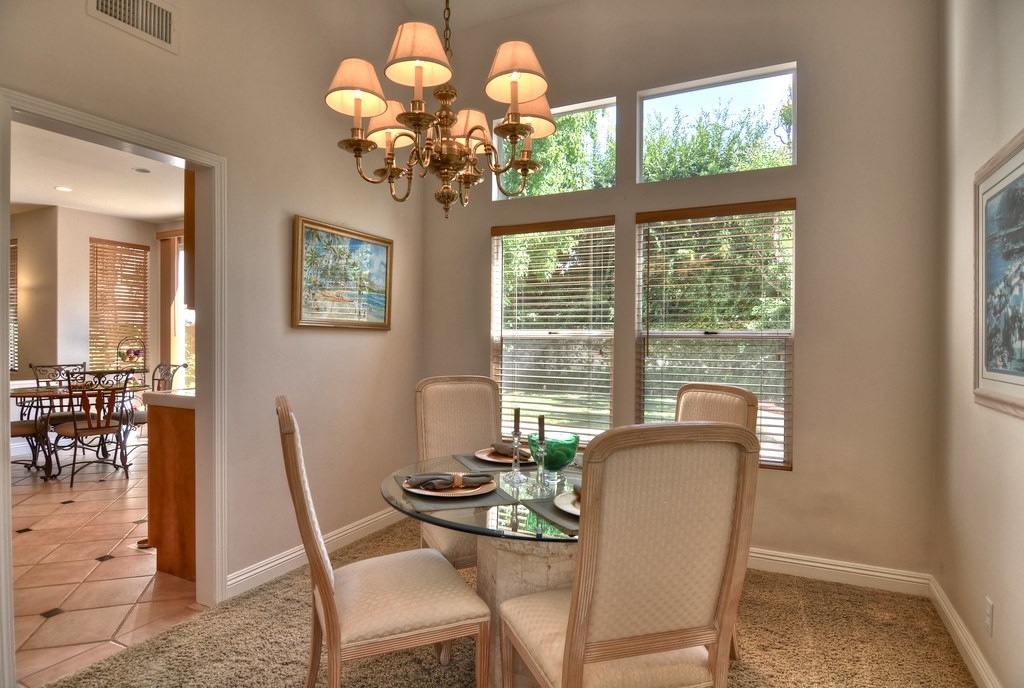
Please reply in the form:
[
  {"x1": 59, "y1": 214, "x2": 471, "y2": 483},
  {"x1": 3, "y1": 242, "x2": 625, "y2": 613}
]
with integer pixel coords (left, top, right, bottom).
[{"x1": 984, "y1": 596, "x2": 994, "y2": 637}]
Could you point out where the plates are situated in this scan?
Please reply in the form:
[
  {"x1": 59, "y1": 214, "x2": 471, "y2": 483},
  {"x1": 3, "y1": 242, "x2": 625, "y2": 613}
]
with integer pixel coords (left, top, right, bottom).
[
  {"x1": 553, "y1": 491, "x2": 581, "y2": 517},
  {"x1": 402, "y1": 470, "x2": 497, "y2": 498},
  {"x1": 474, "y1": 447, "x2": 537, "y2": 464}
]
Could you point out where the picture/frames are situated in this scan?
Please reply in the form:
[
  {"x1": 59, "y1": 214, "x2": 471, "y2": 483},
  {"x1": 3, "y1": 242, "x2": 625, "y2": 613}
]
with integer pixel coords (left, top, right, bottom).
[
  {"x1": 972, "y1": 127, "x2": 1024, "y2": 419},
  {"x1": 290, "y1": 214, "x2": 393, "y2": 331}
]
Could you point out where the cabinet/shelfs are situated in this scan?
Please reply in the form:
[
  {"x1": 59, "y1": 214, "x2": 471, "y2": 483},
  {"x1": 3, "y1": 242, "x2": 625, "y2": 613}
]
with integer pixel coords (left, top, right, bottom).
[{"x1": 142, "y1": 387, "x2": 195, "y2": 585}]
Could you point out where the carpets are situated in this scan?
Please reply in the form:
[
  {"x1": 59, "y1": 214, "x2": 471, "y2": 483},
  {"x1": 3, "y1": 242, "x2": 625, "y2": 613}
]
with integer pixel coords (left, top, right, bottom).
[{"x1": 45, "y1": 513, "x2": 978, "y2": 688}]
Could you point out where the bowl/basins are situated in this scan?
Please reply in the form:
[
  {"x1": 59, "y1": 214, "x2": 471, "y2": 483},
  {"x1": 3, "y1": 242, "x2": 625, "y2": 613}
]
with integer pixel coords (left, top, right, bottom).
[{"x1": 528, "y1": 431, "x2": 579, "y2": 486}]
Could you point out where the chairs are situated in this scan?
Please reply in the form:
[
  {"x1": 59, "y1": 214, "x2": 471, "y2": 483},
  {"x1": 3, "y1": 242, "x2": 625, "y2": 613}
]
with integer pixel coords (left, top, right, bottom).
[
  {"x1": 103, "y1": 363, "x2": 188, "y2": 470},
  {"x1": 415, "y1": 374, "x2": 502, "y2": 567},
  {"x1": 28, "y1": 362, "x2": 86, "y2": 455},
  {"x1": 498, "y1": 420, "x2": 761, "y2": 688},
  {"x1": 675, "y1": 381, "x2": 759, "y2": 434},
  {"x1": 41, "y1": 368, "x2": 135, "y2": 488},
  {"x1": 274, "y1": 395, "x2": 491, "y2": 688}
]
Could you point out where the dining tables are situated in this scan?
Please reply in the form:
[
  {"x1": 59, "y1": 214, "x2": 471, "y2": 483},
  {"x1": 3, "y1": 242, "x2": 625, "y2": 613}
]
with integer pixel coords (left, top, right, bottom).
[
  {"x1": 10, "y1": 384, "x2": 149, "y2": 472},
  {"x1": 380, "y1": 453, "x2": 583, "y2": 688}
]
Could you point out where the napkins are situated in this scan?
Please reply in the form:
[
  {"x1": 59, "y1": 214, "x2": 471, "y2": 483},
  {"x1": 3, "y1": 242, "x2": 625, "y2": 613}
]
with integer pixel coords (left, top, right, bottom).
[{"x1": 408, "y1": 472, "x2": 495, "y2": 492}]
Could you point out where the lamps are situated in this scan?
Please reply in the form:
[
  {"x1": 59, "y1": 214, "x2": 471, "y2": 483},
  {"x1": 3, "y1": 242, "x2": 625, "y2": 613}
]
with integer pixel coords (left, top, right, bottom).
[{"x1": 323, "y1": 0, "x2": 557, "y2": 218}]
